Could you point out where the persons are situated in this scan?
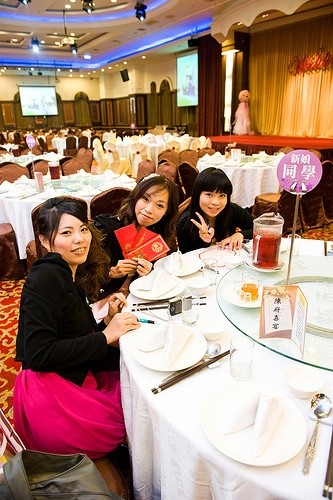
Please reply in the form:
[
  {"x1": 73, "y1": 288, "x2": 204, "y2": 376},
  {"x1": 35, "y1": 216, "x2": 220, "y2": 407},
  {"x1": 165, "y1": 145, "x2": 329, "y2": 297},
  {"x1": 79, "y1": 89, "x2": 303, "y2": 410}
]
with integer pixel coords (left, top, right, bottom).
[
  {"x1": 92, "y1": 176, "x2": 179, "y2": 301},
  {"x1": 12, "y1": 195, "x2": 141, "y2": 459},
  {"x1": 176, "y1": 168, "x2": 255, "y2": 255}
]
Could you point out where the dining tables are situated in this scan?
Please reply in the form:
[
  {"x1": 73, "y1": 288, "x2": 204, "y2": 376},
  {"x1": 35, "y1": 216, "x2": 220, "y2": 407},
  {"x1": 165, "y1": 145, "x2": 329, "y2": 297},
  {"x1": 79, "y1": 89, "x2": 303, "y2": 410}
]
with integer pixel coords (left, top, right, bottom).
[
  {"x1": 1, "y1": 155, "x2": 65, "y2": 168},
  {"x1": 197, "y1": 155, "x2": 289, "y2": 173},
  {"x1": 0, "y1": 173, "x2": 137, "y2": 214},
  {"x1": 118, "y1": 135, "x2": 190, "y2": 148},
  {"x1": 119, "y1": 235, "x2": 333, "y2": 500}
]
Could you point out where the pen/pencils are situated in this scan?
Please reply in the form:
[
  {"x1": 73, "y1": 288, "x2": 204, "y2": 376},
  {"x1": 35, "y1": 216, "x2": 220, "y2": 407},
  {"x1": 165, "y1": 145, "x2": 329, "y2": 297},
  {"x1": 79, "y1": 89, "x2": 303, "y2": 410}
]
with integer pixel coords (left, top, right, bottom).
[
  {"x1": 207, "y1": 219, "x2": 210, "y2": 234},
  {"x1": 137, "y1": 318, "x2": 154, "y2": 325}
]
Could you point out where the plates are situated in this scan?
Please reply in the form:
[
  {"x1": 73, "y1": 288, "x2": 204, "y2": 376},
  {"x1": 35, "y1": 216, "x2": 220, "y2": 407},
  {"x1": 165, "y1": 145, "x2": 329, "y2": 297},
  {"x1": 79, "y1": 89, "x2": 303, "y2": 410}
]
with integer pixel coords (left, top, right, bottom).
[
  {"x1": 154, "y1": 254, "x2": 204, "y2": 276},
  {"x1": 220, "y1": 256, "x2": 287, "y2": 308},
  {"x1": 129, "y1": 275, "x2": 186, "y2": 300},
  {"x1": 199, "y1": 381, "x2": 307, "y2": 467},
  {"x1": 199, "y1": 157, "x2": 281, "y2": 167},
  {"x1": 130, "y1": 326, "x2": 207, "y2": 372},
  {"x1": 214, "y1": 245, "x2": 248, "y2": 263},
  {"x1": 6, "y1": 173, "x2": 133, "y2": 201}
]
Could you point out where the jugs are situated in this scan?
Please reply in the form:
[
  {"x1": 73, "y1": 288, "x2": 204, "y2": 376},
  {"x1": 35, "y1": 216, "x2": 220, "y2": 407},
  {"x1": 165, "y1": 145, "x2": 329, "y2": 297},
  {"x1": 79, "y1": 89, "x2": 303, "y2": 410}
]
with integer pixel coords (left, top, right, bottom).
[{"x1": 252, "y1": 212, "x2": 284, "y2": 269}]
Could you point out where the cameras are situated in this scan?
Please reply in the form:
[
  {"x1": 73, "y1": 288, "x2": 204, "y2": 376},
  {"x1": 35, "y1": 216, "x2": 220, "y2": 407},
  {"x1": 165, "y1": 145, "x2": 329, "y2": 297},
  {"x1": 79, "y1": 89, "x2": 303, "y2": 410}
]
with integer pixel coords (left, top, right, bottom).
[{"x1": 169, "y1": 295, "x2": 192, "y2": 316}]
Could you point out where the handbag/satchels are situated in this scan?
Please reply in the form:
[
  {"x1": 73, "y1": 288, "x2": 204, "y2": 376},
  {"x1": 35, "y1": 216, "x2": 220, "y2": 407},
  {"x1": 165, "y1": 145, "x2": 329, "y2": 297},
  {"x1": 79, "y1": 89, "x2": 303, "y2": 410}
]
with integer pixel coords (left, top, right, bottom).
[{"x1": 0, "y1": 449, "x2": 123, "y2": 500}]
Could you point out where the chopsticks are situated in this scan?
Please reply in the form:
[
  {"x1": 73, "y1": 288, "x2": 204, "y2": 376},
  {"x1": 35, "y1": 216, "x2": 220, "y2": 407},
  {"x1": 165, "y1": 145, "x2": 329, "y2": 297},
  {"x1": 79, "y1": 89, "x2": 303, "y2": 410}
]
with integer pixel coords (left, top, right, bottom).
[
  {"x1": 321, "y1": 427, "x2": 333, "y2": 499},
  {"x1": 132, "y1": 296, "x2": 207, "y2": 312},
  {"x1": 150, "y1": 348, "x2": 237, "y2": 394}
]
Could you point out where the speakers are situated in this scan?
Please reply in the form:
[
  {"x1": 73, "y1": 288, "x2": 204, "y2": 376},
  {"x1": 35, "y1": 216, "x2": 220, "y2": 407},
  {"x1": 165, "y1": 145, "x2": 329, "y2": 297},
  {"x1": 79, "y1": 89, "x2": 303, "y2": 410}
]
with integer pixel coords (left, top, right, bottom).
[
  {"x1": 188, "y1": 39, "x2": 198, "y2": 47},
  {"x1": 120, "y1": 68, "x2": 129, "y2": 82}
]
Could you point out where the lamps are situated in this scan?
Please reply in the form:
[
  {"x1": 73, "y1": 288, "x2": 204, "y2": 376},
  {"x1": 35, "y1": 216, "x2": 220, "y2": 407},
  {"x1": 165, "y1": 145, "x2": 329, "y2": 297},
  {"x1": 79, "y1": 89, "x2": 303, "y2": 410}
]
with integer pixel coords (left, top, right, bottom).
[{"x1": 0, "y1": 0, "x2": 148, "y2": 55}]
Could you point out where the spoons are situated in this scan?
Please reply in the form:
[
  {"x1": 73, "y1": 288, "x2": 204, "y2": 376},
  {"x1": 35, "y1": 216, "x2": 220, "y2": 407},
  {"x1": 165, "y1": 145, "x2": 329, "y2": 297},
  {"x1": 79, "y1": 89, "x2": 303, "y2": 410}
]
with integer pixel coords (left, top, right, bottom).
[{"x1": 301, "y1": 393, "x2": 333, "y2": 476}]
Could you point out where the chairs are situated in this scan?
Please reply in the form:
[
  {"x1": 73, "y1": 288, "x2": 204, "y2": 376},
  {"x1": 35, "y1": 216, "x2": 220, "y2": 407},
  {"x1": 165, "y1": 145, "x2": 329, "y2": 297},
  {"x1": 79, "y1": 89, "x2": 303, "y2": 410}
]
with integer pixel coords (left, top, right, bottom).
[{"x1": 0, "y1": 126, "x2": 333, "y2": 500}]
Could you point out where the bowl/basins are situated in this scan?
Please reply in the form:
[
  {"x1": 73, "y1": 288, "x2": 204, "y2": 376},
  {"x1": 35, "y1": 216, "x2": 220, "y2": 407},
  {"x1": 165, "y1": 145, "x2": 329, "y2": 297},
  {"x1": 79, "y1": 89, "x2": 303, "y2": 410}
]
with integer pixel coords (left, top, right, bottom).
[
  {"x1": 197, "y1": 315, "x2": 228, "y2": 341},
  {"x1": 283, "y1": 367, "x2": 325, "y2": 400},
  {"x1": 222, "y1": 255, "x2": 243, "y2": 268},
  {"x1": 186, "y1": 277, "x2": 210, "y2": 295}
]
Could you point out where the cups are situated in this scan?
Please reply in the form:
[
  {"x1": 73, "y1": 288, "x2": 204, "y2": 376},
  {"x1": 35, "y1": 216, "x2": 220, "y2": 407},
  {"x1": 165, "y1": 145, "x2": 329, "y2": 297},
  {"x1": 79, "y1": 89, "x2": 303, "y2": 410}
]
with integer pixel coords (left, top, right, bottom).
[
  {"x1": 201, "y1": 259, "x2": 218, "y2": 286},
  {"x1": 286, "y1": 233, "x2": 301, "y2": 257},
  {"x1": 229, "y1": 335, "x2": 255, "y2": 379},
  {"x1": 231, "y1": 149, "x2": 241, "y2": 159},
  {"x1": 241, "y1": 151, "x2": 245, "y2": 156},
  {"x1": 49, "y1": 166, "x2": 60, "y2": 179},
  {"x1": 181, "y1": 290, "x2": 200, "y2": 326}
]
